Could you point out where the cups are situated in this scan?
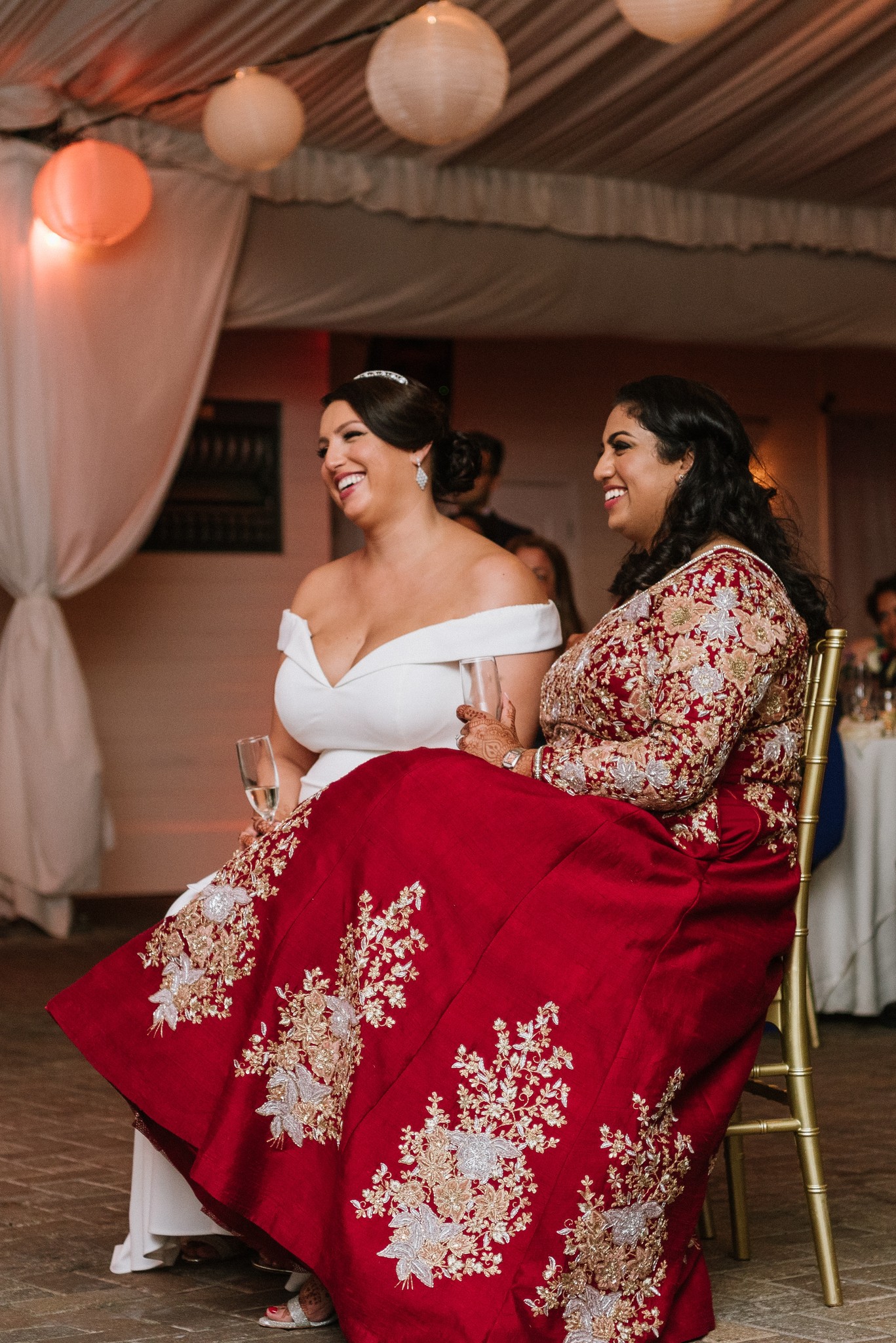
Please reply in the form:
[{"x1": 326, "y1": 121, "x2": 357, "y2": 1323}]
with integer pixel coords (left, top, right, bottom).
[
  {"x1": 236, "y1": 735, "x2": 280, "y2": 824},
  {"x1": 459, "y1": 655, "x2": 502, "y2": 722},
  {"x1": 843, "y1": 653, "x2": 894, "y2": 738}
]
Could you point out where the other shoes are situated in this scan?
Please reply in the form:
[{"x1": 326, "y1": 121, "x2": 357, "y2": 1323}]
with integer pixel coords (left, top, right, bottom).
[
  {"x1": 182, "y1": 1235, "x2": 245, "y2": 1261},
  {"x1": 252, "y1": 1238, "x2": 300, "y2": 1272}
]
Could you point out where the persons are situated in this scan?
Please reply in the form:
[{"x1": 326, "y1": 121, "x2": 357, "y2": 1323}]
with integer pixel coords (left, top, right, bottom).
[
  {"x1": 861, "y1": 572, "x2": 896, "y2": 689},
  {"x1": 440, "y1": 426, "x2": 537, "y2": 549},
  {"x1": 50, "y1": 373, "x2": 836, "y2": 1343},
  {"x1": 97, "y1": 370, "x2": 564, "y2": 1277},
  {"x1": 504, "y1": 533, "x2": 586, "y2": 642}
]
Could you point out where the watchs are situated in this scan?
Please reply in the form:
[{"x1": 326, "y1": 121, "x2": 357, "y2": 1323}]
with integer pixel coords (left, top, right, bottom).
[{"x1": 502, "y1": 745, "x2": 526, "y2": 772}]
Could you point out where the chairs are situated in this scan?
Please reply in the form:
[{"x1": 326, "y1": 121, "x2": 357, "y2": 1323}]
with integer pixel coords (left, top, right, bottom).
[{"x1": 703, "y1": 624, "x2": 849, "y2": 1309}]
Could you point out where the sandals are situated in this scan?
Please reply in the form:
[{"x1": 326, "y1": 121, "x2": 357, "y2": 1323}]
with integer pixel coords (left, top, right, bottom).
[{"x1": 258, "y1": 1275, "x2": 337, "y2": 1328}]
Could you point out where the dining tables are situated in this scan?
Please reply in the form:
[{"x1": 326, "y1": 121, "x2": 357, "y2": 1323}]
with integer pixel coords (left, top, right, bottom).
[{"x1": 806, "y1": 709, "x2": 895, "y2": 1020}]
